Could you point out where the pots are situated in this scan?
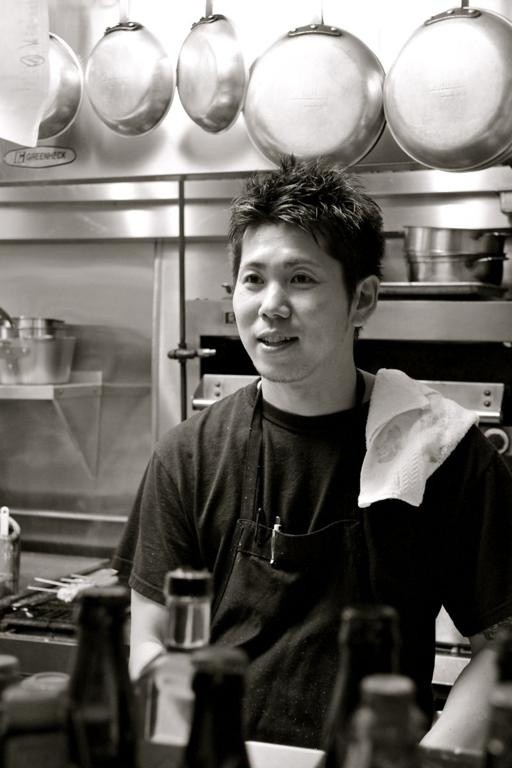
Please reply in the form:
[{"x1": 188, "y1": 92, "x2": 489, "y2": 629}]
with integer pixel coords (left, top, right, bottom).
[
  {"x1": 0, "y1": 306, "x2": 78, "y2": 385},
  {"x1": 85, "y1": 2, "x2": 176, "y2": 140},
  {"x1": 380, "y1": 1, "x2": 512, "y2": 175},
  {"x1": 403, "y1": 222, "x2": 511, "y2": 287},
  {"x1": 37, "y1": 36, "x2": 84, "y2": 142},
  {"x1": 175, "y1": 1, "x2": 249, "y2": 137},
  {"x1": 243, "y1": 9, "x2": 389, "y2": 177}
]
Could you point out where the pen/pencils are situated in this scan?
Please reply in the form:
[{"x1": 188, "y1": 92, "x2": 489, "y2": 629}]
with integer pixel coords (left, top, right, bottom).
[
  {"x1": 269, "y1": 515, "x2": 282, "y2": 564},
  {"x1": 254, "y1": 507, "x2": 262, "y2": 543}
]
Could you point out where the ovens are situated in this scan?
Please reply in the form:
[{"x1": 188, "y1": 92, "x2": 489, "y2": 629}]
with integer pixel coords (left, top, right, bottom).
[{"x1": 180, "y1": 296, "x2": 511, "y2": 468}]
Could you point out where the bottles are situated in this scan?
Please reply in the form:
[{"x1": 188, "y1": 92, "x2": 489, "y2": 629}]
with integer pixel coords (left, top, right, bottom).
[
  {"x1": 313, "y1": 608, "x2": 398, "y2": 768},
  {"x1": 132, "y1": 566, "x2": 214, "y2": 766},
  {"x1": 180, "y1": 650, "x2": 257, "y2": 768},
  {"x1": 343, "y1": 677, "x2": 428, "y2": 768},
  {"x1": 70, "y1": 583, "x2": 134, "y2": 768}
]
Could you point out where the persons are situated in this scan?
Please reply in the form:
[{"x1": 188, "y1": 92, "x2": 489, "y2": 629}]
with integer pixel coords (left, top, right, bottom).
[{"x1": 108, "y1": 159, "x2": 511, "y2": 752}]
